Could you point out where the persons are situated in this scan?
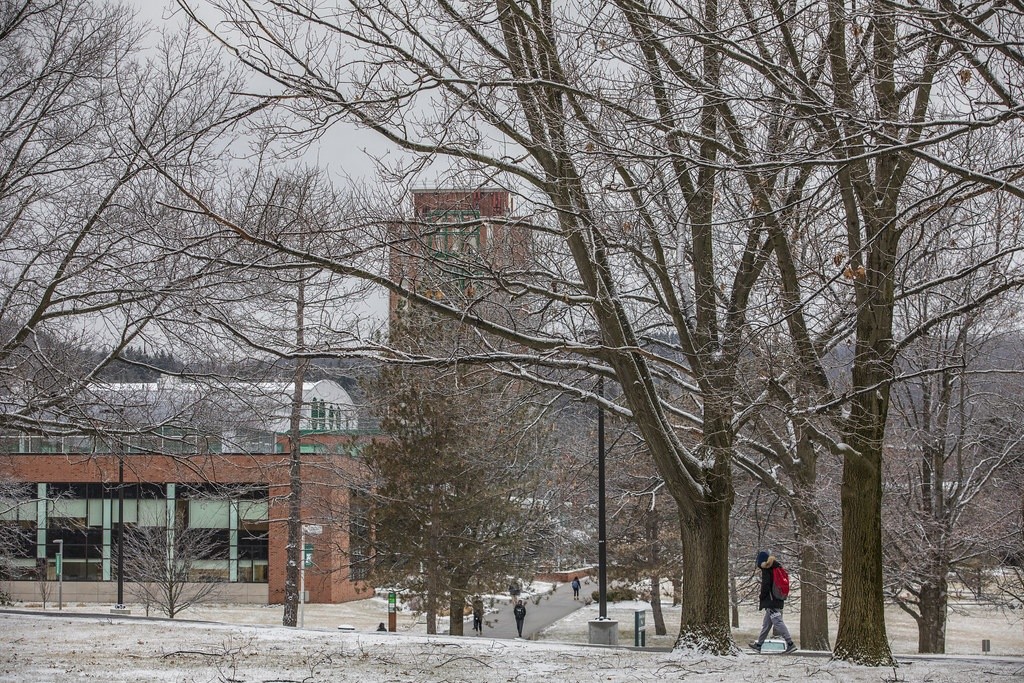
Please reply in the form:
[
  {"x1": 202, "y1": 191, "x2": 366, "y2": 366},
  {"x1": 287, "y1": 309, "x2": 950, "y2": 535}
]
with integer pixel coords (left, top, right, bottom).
[
  {"x1": 514, "y1": 600, "x2": 526, "y2": 638},
  {"x1": 572, "y1": 577, "x2": 581, "y2": 600},
  {"x1": 376, "y1": 623, "x2": 388, "y2": 632},
  {"x1": 748, "y1": 552, "x2": 798, "y2": 656},
  {"x1": 509, "y1": 580, "x2": 519, "y2": 608},
  {"x1": 472, "y1": 596, "x2": 483, "y2": 637}
]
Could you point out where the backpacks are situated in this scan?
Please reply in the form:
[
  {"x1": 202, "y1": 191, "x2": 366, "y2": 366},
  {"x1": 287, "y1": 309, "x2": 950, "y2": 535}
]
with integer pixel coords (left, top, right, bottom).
[
  {"x1": 572, "y1": 581, "x2": 579, "y2": 588},
  {"x1": 772, "y1": 567, "x2": 789, "y2": 599},
  {"x1": 516, "y1": 608, "x2": 524, "y2": 619}
]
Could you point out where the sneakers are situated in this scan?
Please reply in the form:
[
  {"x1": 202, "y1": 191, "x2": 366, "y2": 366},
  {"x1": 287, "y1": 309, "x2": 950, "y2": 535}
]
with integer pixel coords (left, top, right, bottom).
[
  {"x1": 749, "y1": 642, "x2": 761, "y2": 652},
  {"x1": 782, "y1": 644, "x2": 797, "y2": 654}
]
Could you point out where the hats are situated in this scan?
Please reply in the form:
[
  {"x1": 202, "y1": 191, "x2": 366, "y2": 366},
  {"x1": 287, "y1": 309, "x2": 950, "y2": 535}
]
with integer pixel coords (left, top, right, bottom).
[{"x1": 756, "y1": 552, "x2": 768, "y2": 563}]
